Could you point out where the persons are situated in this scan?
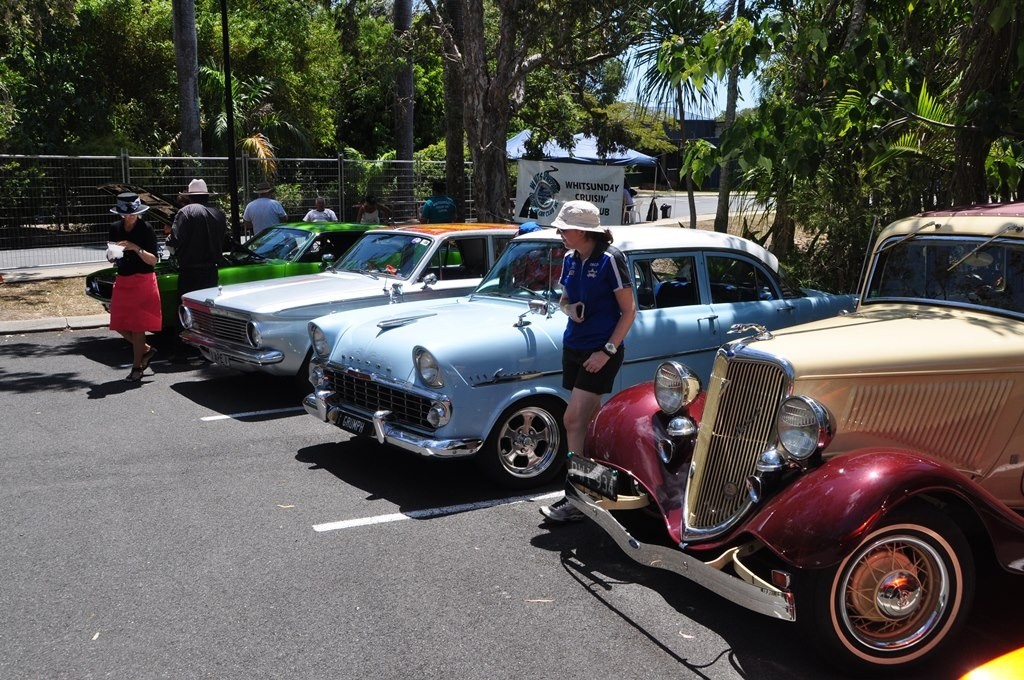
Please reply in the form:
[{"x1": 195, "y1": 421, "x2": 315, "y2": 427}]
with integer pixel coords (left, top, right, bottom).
[
  {"x1": 242, "y1": 182, "x2": 287, "y2": 236},
  {"x1": 620, "y1": 175, "x2": 635, "y2": 222},
  {"x1": 536, "y1": 199, "x2": 635, "y2": 523},
  {"x1": 302, "y1": 197, "x2": 338, "y2": 223},
  {"x1": 355, "y1": 193, "x2": 392, "y2": 225},
  {"x1": 165, "y1": 179, "x2": 224, "y2": 357},
  {"x1": 106, "y1": 193, "x2": 159, "y2": 384},
  {"x1": 416, "y1": 180, "x2": 457, "y2": 225}
]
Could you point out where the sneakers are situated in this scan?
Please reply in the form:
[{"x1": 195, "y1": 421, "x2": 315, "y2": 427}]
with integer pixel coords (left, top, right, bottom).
[{"x1": 539, "y1": 497, "x2": 586, "y2": 524}]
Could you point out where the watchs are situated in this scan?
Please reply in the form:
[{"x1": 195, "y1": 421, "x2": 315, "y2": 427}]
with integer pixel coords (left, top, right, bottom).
[{"x1": 605, "y1": 342, "x2": 618, "y2": 355}]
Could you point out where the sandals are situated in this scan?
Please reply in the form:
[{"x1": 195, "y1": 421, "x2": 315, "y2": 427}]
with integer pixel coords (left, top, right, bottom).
[
  {"x1": 141, "y1": 348, "x2": 157, "y2": 370},
  {"x1": 126, "y1": 364, "x2": 143, "y2": 381}
]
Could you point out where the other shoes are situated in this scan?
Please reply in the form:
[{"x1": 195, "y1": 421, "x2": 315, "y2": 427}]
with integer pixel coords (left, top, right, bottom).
[{"x1": 169, "y1": 356, "x2": 188, "y2": 363}]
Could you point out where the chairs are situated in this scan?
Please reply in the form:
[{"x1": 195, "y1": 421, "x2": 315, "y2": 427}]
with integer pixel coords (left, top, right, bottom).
[
  {"x1": 623, "y1": 200, "x2": 643, "y2": 224},
  {"x1": 654, "y1": 281, "x2": 749, "y2": 306},
  {"x1": 637, "y1": 286, "x2": 652, "y2": 310},
  {"x1": 449, "y1": 252, "x2": 486, "y2": 279}
]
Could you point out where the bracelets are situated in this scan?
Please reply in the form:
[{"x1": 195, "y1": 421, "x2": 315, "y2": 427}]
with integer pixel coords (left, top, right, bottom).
[
  {"x1": 563, "y1": 303, "x2": 569, "y2": 316},
  {"x1": 135, "y1": 247, "x2": 142, "y2": 255},
  {"x1": 601, "y1": 347, "x2": 612, "y2": 358}
]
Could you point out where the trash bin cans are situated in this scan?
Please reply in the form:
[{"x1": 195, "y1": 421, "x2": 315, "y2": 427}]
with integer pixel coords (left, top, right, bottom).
[{"x1": 662, "y1": 205, "x2": 671, "y2": 218}]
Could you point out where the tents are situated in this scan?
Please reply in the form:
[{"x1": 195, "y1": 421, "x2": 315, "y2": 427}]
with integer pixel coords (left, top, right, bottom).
[{"x1": 505, "y1": 125, "x2": 662, "y2": 222}]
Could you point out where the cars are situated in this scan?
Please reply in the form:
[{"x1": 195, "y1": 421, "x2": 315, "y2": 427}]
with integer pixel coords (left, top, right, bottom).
[
  {"x1": 176, "y1": 221, "x2": 546, "y2": 397},
  {"x1": 562, "y1": 201, "x2": 1023, "y2": 680},
  {"x1": 83, "y1": 223, "x2": 464, "y2": 361},
  {"x1": 299, "y1": 225, "x2": 859, "y2": 488}
]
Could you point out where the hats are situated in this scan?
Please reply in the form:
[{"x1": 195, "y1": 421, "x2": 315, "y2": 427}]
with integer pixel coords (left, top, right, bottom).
[
  {"x1": 178, "y1": 179, "x2": 212, "y2": 195},
  {"x1": 551, "y1": 200, "x2": 606, "y2": 233},
  {"x1": 253, "y1": 182, "x2": 272, "y2": 193},
  {"x1": 110, "y1": 193, "x2": 149, "y2": 214},
  {"x1": 515, "y1": 222, "x2": 541, "y2": 237}
]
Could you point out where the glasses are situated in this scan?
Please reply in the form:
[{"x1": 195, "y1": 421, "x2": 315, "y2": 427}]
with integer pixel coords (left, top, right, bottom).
[{"x1": 558, "y1": 229, "x2": 565, "y2": 233}]
